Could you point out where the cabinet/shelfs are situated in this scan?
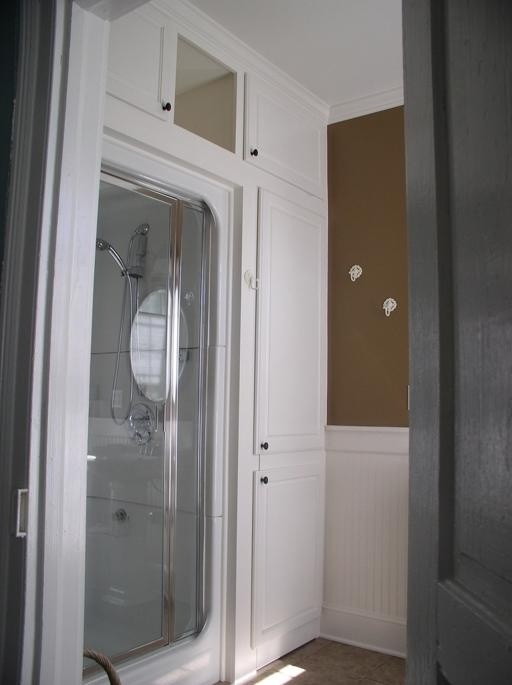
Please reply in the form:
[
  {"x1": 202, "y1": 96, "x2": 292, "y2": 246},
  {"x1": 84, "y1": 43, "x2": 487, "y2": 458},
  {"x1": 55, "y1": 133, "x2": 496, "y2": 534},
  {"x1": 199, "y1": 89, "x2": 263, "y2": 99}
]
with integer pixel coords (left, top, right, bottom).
[
  {"x1": 174, "y1": 33, "x2": 330, "y2": 200},
  {"x1": 229, "y1": 187, "x2": 323, "y2": 470},
  {"x1": 228, "y1": 453, "x2": 326, "y2": 685},
  {"x1": 106, "y1": 0, "x2": 178, "y2": 122}
]
